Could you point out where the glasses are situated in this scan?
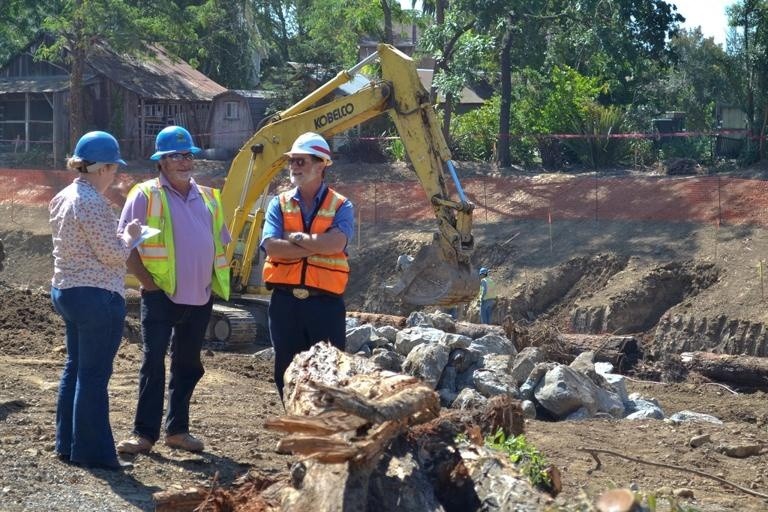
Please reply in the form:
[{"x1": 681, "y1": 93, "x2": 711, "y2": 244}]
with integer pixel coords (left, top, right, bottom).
[
  {"x1": 168, "y1": 152, "x2": 195, "y2": 161},
  {"x1": 288, "y1": 157, "x2": 310, "y2": 167}
]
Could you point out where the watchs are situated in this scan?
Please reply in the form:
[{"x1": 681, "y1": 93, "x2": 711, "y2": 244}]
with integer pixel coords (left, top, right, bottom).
[{"x1": 293, "y1": 234, "x2": 303, "y2": 246}]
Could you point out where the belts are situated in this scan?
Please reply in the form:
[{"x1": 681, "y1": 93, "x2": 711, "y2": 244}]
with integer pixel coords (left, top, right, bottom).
[{"x1": 276, "y1": 284, "x2": 321, "y2": 301}]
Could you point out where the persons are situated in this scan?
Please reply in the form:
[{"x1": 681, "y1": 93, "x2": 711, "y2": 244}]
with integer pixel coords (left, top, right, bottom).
[
  {"x1": 48, "y1": 130, "x2": 143, "y2": 467},
  {"x1": 115, "y1": 125, "x2": 233, "y2": 454},
  {"x1": 478, "y1": 267, "x2": 497, "y2": 324},
  {"x1": 260, "y1": 131, "x2": 353, "y2": 452}
]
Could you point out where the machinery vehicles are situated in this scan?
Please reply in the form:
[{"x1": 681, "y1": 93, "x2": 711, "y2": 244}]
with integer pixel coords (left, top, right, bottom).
[{"x1": 124, "y1": 44, "x2": 479, "y2": 350}]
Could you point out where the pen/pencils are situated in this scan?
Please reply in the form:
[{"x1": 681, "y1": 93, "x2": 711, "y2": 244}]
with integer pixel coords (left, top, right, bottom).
[{"x1": 123, "y1": 220, "x2": 128, "y2": 226}]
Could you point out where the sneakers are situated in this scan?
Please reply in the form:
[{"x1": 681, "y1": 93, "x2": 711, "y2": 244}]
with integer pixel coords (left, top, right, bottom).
[
  {"x1": 275, "y1": 438, "x2": 292, "y2": 455},
  {"x1": 117, "y1": 433, "x2": 152, "y2": 454},
  {"x1": 164, "y1": 432, "x2": 204, "y2": 452}
]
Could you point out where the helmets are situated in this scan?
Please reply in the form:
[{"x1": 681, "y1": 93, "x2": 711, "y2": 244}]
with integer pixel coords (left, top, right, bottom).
[
  {"x1": 74, "y1": 131, "x2": 128, "y2": 167},
  {"x1": 150, "y1": 125, "x2": 202, "y2": 161},
  {"x1": 479, "y1": 268, "x2": 488, "y2": 274},
  {"x1": 283, "y1": 132, "x2": 333, "y2": 167}
]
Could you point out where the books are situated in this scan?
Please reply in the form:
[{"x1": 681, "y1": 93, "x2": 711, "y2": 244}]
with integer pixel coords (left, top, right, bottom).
[{"x1": 130, "y1": 224, "x2": 161, "y2": 249}]
